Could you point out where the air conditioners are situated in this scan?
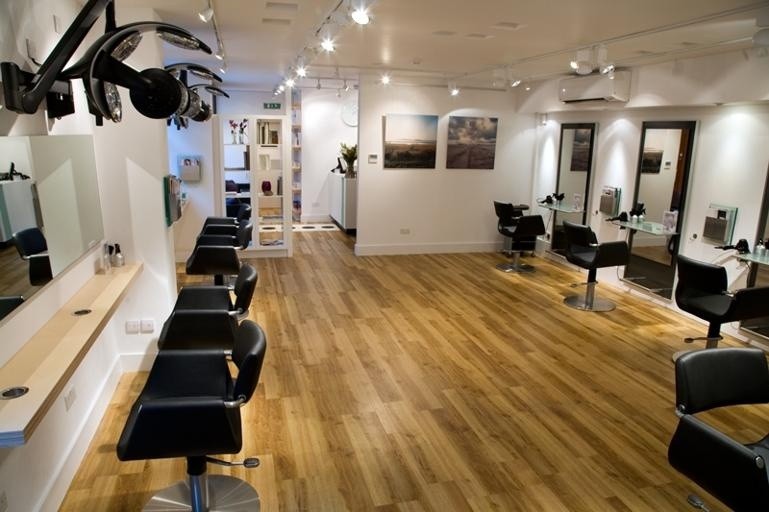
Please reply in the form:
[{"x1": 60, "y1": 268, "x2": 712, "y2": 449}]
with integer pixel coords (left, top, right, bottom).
[{"x1": 558, "y1": 69, "x2": 631, "y2": 103}]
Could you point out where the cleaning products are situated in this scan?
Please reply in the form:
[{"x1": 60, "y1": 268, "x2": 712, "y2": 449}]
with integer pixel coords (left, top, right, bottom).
[
  {"x1": 638, "y1": 207, "x2": 646, "y2": 223},
  {"x1": 552, "y1": 192, "x2": 557, "y2": 205},
  {"x1": 630, "y1": 206, "x2": 638, "y2": 224}
]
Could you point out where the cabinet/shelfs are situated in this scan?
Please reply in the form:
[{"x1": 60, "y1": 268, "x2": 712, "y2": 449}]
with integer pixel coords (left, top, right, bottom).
[{"x1": 219, "y1": 114, "x2": 293, "y2": 258}]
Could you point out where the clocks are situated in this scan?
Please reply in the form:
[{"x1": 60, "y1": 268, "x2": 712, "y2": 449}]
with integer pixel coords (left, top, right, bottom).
[{"x1": 341, "y1": 101, "x2": 358, "y2": 127}]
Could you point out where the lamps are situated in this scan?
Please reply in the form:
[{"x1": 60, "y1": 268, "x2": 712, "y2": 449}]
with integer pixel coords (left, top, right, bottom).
[
  {"x1": 508, "y1": 66, "x2": 521, "y2": 88},
  {"x1": 215, "y1": 49, "x2": 224, "y2": 60},
  {"x1": 447, "y1": 82, "x2": 460, "y2": 97},
  {"x1": 570, "y1": 43, "x2": 616, "y2": 76},
  {"x1": 273, "y1": 8, "x2": 370, "y2": 97},
  {"x1": 219, "y1": 65, "x2": 227, "y2": 74},
  {"x1": 198, "y1": 7, "x2": 214, "y2": 23}
]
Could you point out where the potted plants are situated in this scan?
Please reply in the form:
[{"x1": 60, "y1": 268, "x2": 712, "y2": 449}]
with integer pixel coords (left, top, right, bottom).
[{"x1": 340, "y1": 142, "x2": 357, "y2": 178}]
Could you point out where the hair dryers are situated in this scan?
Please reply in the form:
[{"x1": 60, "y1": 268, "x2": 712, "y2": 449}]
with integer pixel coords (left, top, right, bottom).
[
  {"x1": 606, "y1": 211, "x2": 628, "y2": 229},
  {"x1": 715, "y1": 238, "x2": 750, "y2": 263},
  {"x1": 541, "y1": 194, "x2": 553, "y2": 211}
]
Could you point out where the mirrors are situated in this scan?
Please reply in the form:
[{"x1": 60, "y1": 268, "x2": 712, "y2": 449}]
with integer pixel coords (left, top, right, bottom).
[
  {"x1": 737, "y1": 163, "x2": 769, "y2": 346},
  {"x1": 0, "y1": 135, "x2": 104, "y2": 322},
  {"x1": 619, "y1": 120, "x2": 700, "y2": 305},
  {"x1": 550, "y1": 120, "x2": 599, "y2": 257}
]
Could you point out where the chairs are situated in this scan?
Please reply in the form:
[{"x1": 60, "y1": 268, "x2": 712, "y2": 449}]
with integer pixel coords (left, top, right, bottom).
[
  {"x1": 12, "y1": 227, "x2": 52, "y2": 286},
  {"x1": 494, "y1": 201, "x2": 546, "y2": 274},
  {"x1": 157, "y1": 262, "x2": 258, "y2": 386},
  {"x1": 563, "y1": 219, "x2": 629, "y2": 312},
  {"x1": 186, "y1": 203, "x2": 254, "y2": 289},
  {"x1": 0, "y1": 295, "x2": 25, "y2": 322},
  {"x1": 671, "y1": 254, "x2": 769, "y2": 364},
  {"x1": 117, "y1": 320, "x2": 266, "y2": 511},
  {"x1": 667, "y1": 348, "x2": 769, "y2": 512}
]
